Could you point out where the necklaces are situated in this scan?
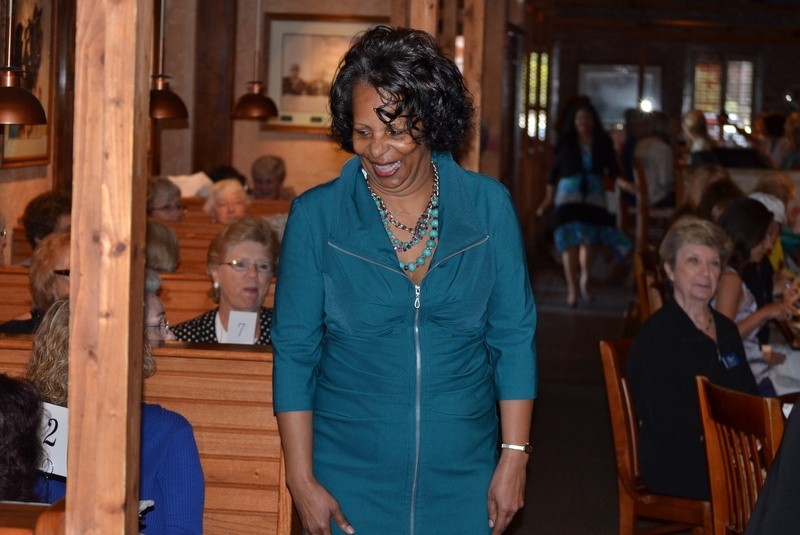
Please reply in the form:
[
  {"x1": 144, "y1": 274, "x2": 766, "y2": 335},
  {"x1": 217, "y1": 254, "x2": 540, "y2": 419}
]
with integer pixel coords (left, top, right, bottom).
[{"x1": 362, "y1": 160, "x2": 440, "y2": 272}]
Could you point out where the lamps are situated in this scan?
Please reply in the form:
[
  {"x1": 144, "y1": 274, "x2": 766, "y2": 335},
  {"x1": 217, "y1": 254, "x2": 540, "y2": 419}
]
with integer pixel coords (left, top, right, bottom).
[
  {"x1": 0, "y1": 0, "x2": 46, "y2": 125},
  {"x1": 229, "y1": 0, "x2": 278, "y2": 119},
  {"x1": 149, "y1": 1, "x2": 189, "y2": 121}
]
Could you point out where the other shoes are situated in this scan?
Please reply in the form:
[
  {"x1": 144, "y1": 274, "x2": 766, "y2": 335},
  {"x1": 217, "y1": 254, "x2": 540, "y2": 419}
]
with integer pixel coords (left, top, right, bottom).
[
  {"x1": 580, "y1": 281, "x2": 592, "y2": 305},
  {"x1": 567, "y1": 290, "x2": 577, "y2": 306}
]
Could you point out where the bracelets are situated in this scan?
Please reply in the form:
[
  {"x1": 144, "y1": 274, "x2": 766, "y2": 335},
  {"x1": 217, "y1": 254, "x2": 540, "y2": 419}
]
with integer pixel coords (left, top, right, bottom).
[{"x1": 502, "y1": 443, "x2": 534, "y2": 454}]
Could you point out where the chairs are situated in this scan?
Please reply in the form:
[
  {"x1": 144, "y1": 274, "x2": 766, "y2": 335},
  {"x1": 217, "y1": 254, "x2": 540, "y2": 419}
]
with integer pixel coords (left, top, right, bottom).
[{"x1": 597, "y1": 159, "x2": 786, "y2": 534}]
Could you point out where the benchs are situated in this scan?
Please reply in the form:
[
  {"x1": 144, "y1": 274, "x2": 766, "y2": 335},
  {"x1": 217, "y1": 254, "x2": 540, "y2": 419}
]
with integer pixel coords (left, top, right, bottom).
[{"x1": 0, "y1": 193, "x2": 298, "y2": 534}]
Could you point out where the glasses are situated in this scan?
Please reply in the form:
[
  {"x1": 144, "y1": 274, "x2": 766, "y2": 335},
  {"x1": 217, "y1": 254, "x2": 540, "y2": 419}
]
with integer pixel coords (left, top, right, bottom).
[
  {"x1": 146, "y1": 317, "x2": 170, "y2": 334},
  {"x1": 153, "y1": 201, "x2": 187, "y2": 213},
  {"x1": 220, "y1": 258, "x2": 277, "y2": 272}
]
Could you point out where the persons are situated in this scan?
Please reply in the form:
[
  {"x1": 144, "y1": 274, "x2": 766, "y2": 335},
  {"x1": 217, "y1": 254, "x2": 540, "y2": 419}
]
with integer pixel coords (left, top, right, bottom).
[
  {"x1": 0, "y1": 373, "x2": 54, "y2": 503},
  {"x1": 25, "y1": 293, "x2": 206, "y2": 535},
  {"x1": 270, "y1": 26, "x2": 537, "y2": 535},
  {"x1": 143, "y1": 268, "x2": 168, "y2": 340},
  {"x1": 622, "y1": 216, "x2": 750, "y2": 502},
  {"x1": 536, "y1": 93, "x2": 800, "y2": 535},
  {"x1": 0, "y1": 231, "x2": 71, "y2": 334},
  {"x1": 0, "y1": 154, "x2": 296, "y2": 272},
  {"x1": 170, "y1": 216, "x2": 281, "y2": 346}
]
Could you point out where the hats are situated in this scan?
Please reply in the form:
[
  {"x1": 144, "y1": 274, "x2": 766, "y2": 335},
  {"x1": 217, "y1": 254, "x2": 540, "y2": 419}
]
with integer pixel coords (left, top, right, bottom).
[{"x1": 746, "y1": 192, "x2": 788, "y2": 223}]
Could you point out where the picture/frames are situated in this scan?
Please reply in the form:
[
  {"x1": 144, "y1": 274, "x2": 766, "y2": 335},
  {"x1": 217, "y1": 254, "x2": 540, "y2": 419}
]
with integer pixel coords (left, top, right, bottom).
[
  {"x1": 0, "y1": 0, "x2": 51, "y2": 169},
  {"x1": 257, "y1": 11, "x2": 389, "y2": 132}
]
